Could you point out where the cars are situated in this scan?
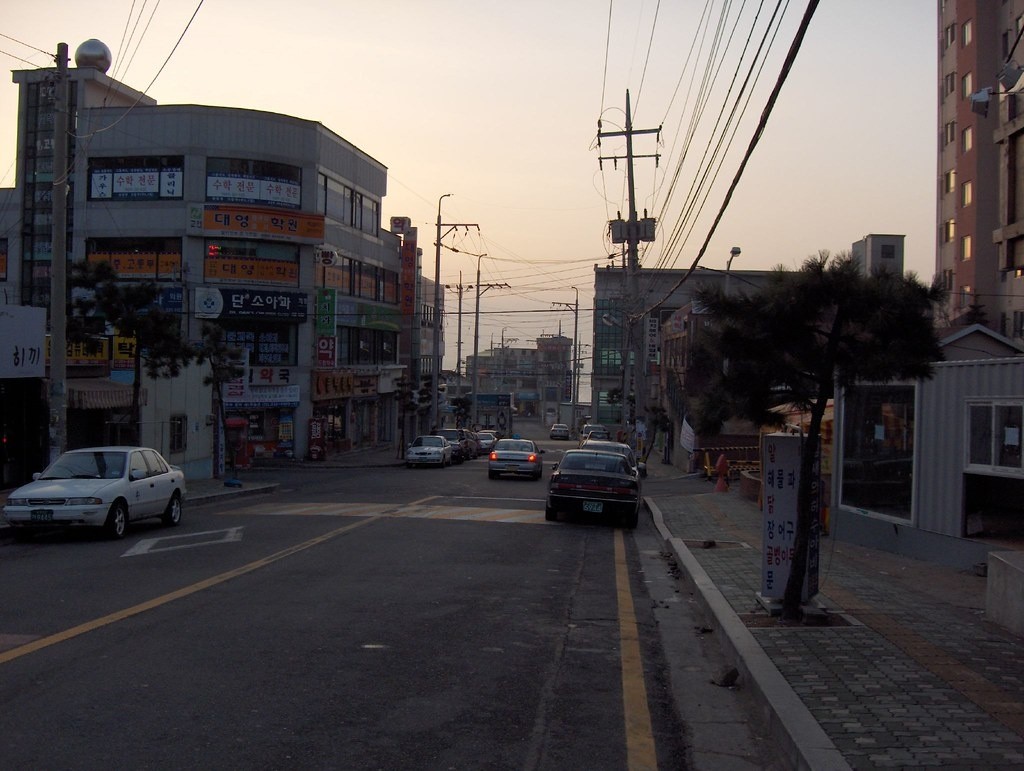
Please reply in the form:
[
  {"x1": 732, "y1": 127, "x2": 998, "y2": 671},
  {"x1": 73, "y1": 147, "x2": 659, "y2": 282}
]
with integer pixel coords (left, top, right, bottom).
[
  {"x1": 429, "y1": 429, "x2": 465, "y2": 465},
  {"x1": 549, "y1": 424, "x2": 569, "y2": 440},
  {"x1": 1, "y1": 444, "x2": 187, "y2": 537},
  {"x1": 488, "y1": 433, "x2": 546, "y2": 481},
  {"x1": 578, "y1": 438, "x2": 640, "y2": 488},
  {"x1": 454, "y1": 428, "x2": 502, "y2": 461},
  {"x1": 586, "y1": 431, "x2": 613, "y2": 441},
  {"x1": 406, "y1": 435, "x2": 452, "y2": 468},
  {"x1": 580, "y1": 425, "x2": 606, "y2": 439},
  {"x1": 544, "y1": 448, "x2": 641, "y2": 531}
]
